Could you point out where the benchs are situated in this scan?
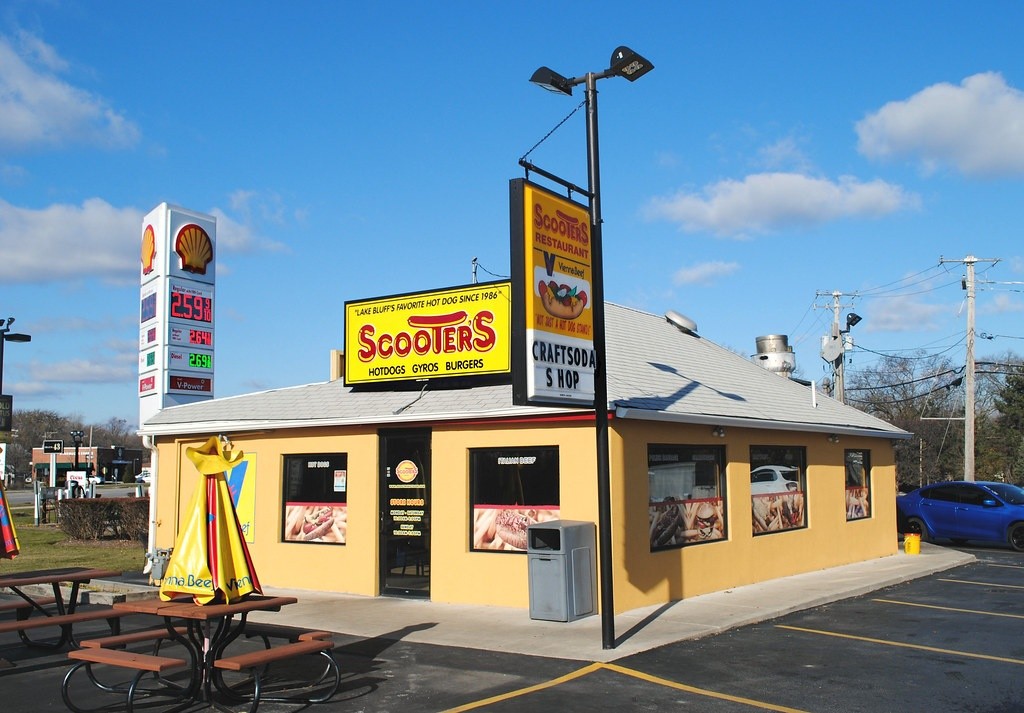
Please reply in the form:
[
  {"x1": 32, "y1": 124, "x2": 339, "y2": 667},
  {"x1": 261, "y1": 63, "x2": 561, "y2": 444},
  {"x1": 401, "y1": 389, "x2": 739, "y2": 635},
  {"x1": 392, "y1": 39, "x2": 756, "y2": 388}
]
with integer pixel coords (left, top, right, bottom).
[
  {"x1": 62, "y1": 647, "x2": 186, "y2": 713},
  {"x1": 222, "y1": 625, "x2": 332, "y2": 698},
  {"x1": 80, "y1": 625, "x2": 201, "y2": 702},
  {"x1": 0, "y1": 609, "x2": 139, "y2": 659},
  {"x1": 213, "y1": 639, "x2": 340, "y2": 713},
  {"x1": 0, "y1": 597, "x2": 64, "y2": 652}
]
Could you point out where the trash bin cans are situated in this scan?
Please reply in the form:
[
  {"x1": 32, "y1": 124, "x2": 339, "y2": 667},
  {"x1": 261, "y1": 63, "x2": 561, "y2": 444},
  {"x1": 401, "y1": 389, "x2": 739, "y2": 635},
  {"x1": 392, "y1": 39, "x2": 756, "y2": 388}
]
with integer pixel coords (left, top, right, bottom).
[{"x1": 527, "y1": 520, "x2": 598, "y2": 624}]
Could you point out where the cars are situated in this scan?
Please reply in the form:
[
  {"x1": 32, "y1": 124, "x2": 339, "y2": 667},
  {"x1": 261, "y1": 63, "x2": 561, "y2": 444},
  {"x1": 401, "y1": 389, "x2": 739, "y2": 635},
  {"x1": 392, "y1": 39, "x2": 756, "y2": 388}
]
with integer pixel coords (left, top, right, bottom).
[
  {"x1": 87, "y1": 475, "x2": 104, "y2": 485},
  {"x1": 136, "y1": 471, "x2": 150, "y2": 483},
  {"x1": 896, "y1": 481, "x2": 1024, "y2": 551},
  {"x1": 751, "y1": 466, "x2": 798, "y2": 494},
  {"x1": 25, "y1": 473, "x2": 32, "y2": 484}
]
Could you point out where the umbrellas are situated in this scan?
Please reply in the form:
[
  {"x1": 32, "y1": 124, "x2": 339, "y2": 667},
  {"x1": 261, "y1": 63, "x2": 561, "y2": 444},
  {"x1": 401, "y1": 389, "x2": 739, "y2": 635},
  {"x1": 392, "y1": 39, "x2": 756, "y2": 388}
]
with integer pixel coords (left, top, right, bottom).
[
  {"x1": 0, "y1": 480, "x2": 20, "y2": 561},
  {"x1": 158, "y1": 433, "x2": 264, "y2": 686}
]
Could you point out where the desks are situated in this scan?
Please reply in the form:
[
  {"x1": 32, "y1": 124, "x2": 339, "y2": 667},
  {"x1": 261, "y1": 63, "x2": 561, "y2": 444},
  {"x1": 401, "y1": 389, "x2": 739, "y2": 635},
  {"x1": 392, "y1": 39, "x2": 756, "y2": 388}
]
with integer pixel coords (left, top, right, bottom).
[
  {"x1": 0, "y1": 567, "x2": 129, "y2": 650},
  {"x1": 113, "y1": 591, "x2": 297, "y2": 705}
]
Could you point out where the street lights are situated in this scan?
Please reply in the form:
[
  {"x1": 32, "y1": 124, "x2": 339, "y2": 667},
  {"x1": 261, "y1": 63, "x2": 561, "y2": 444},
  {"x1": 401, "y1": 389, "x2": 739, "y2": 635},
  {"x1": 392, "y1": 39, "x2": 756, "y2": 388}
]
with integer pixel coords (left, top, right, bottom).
[
  {"x1": 529, "y1": 46, "x2": 656, "y2": 649},
  {"x1": 70, "y1": 431, "x2": 84, "y2": 471},
  {"x1": 838, "y1": 314, "x2": 862, "y2": 403},
  {"x1": 0, "y1": 317, "x2": 31, "y2": 395}
]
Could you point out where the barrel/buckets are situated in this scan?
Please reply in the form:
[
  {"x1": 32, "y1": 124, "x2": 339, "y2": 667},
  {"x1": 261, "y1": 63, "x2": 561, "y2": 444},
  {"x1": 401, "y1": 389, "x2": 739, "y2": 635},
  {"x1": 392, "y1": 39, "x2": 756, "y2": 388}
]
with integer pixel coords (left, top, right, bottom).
[{"x1": 904, "y1": 533, "x2": 921, "y2": 554}]
